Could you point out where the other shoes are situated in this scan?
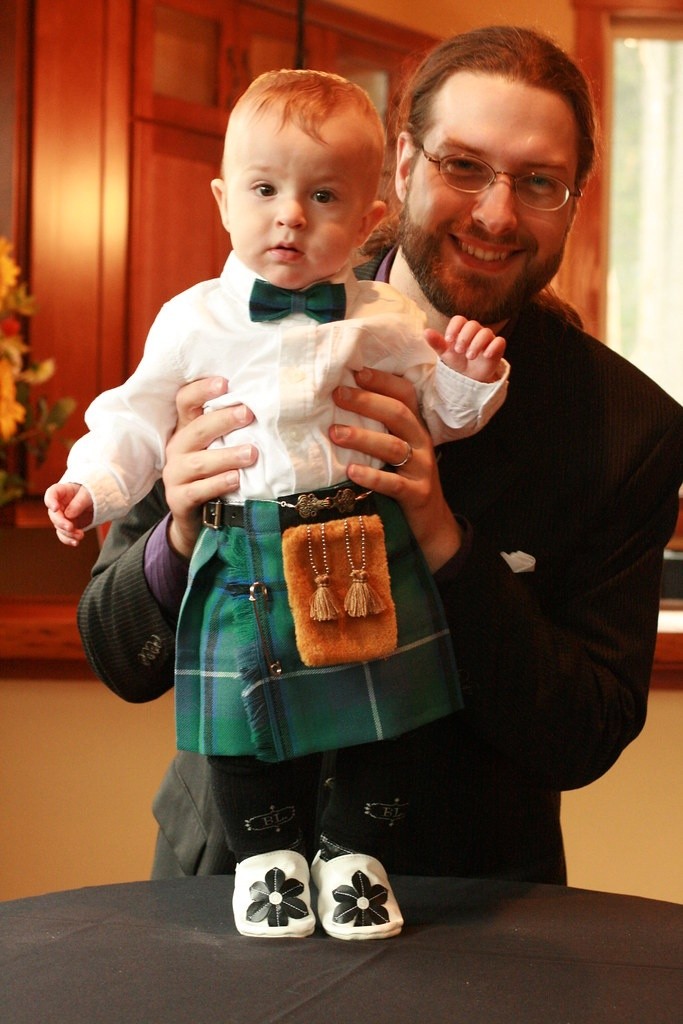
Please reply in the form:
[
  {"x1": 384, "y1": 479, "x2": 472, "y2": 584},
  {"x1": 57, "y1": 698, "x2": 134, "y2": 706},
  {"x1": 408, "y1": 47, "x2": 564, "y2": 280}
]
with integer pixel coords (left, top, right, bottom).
[
  {"x1": 311, "y1": 850, "x2": 404, "y2": 940},
  {"x1": 232, "y1": 850, "x2": 316, "y2": 938}
]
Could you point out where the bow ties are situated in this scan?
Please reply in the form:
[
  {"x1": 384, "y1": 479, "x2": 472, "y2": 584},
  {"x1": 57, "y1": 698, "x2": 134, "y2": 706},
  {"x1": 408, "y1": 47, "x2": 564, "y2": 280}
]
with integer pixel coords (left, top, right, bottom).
[{"x1": 249, "y1": 278, "x2": 346, "y2": 323}]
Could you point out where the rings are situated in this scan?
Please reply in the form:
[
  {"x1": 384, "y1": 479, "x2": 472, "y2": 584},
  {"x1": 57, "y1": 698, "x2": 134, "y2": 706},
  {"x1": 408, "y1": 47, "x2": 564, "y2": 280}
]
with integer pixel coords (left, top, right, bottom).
[{"x1": 393, "y1": 445, "x2": 413, "y2": 466}]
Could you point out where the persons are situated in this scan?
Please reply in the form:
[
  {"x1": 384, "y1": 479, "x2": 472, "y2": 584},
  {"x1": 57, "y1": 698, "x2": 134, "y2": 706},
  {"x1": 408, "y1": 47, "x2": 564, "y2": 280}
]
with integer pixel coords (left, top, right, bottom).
[
  {"x1": 44, "y1": 67, "x2": 511, "y2": 941},
  {"x1": 75, "y1": 24, "x2": 683, "y2": 882}
]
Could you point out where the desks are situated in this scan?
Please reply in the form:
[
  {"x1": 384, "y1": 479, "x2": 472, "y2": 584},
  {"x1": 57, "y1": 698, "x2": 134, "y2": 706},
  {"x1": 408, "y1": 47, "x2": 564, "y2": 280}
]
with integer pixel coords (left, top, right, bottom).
[{"x1": 0, "y1": 874, "x2": 683, "y2": 1024}]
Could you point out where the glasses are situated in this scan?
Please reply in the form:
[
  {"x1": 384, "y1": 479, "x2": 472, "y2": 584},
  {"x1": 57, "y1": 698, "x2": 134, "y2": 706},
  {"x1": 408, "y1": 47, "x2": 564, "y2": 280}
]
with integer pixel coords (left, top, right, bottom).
[{"x1": 421, "y1": 142, "x2": 583, "y2": 211}]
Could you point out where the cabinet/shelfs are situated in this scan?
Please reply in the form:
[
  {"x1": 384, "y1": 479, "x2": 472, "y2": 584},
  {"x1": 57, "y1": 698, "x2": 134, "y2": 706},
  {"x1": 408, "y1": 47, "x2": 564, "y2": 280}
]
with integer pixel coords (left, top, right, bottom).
[{"x1": 12, "y1": 0, "x2": 446, "y2": 528}]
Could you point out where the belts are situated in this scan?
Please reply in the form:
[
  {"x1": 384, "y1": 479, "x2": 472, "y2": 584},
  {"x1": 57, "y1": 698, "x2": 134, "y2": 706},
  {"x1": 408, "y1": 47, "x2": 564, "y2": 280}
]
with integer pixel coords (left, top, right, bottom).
[{"x1": 202, "y1": 488, "x2": 380, "y2": 530}]
